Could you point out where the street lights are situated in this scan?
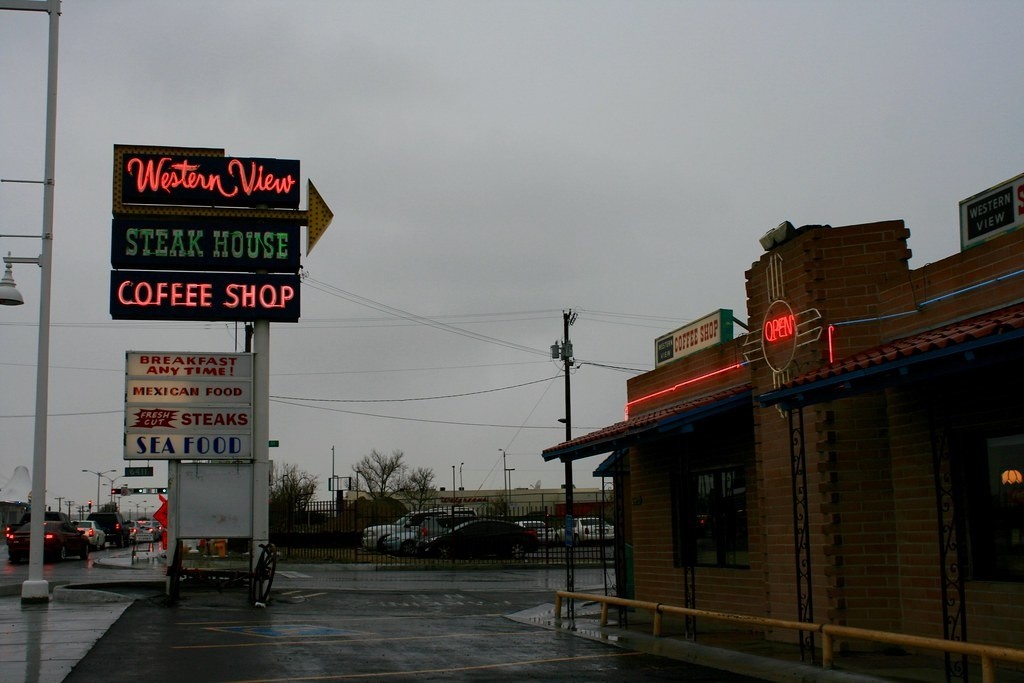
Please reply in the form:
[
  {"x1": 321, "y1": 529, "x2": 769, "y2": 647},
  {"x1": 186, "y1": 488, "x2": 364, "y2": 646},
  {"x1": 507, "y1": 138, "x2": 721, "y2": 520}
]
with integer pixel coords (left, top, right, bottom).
[
  {"x1": 80, "y1": 468, "x2": 157, "y2": 521},
  {"x1": 451, "y1": 445, "x2": 516, "y2": 501},
  {"x1": 0, "y1": 0, "x2": 63, "y2": 606}
]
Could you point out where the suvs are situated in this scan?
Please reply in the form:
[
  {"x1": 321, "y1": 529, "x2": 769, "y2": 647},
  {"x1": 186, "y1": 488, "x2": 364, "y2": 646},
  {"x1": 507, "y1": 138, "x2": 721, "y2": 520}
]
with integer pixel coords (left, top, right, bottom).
[{"x1": 362, "y1": 507, "x2": 476, "y2": 554}]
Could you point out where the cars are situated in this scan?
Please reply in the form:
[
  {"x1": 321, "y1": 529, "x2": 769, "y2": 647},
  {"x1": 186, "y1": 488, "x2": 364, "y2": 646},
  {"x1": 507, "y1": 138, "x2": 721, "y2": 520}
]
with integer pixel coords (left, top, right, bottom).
[
  {"x1": 7, "y1": 511, "x2": 165, "y2": 562},
  {"x1": 417, "y1": 518, "x2": 539, "y2": 560},
  {"x1": 515, "y1": 521, "x2": 556, "y2": 547},
  {"x1": 383, "y1": 514, "x2": 478, "y2": 557},
  {"x1": 557, "y1": 518, "x2": 616, "y2": 548}
]
